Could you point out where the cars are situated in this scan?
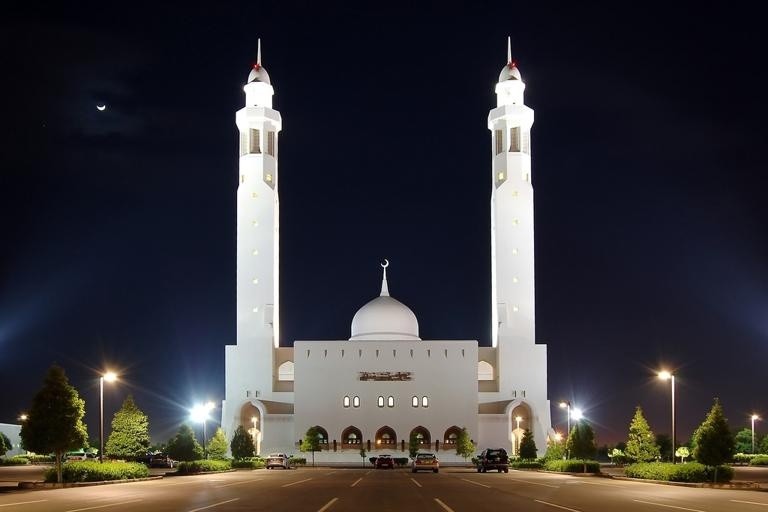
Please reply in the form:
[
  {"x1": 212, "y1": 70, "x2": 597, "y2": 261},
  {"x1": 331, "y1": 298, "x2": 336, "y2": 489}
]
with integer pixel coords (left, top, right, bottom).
[
  {"x1": 375, "y1": 455, "x2": 394, "y2": 469},
  {"x1": 477, "y1": 448, "x2": 508, "y2": 473},
  {"x1": 267, "y1": 453, "x2": 287, "y2": 469},
  {"x1": 412, "y1": 453, "x2": 439, "y2": 473},
  {"x1": 150, "y1": 453, "x2": 173, "y2": 468},
  {"x1": 67, "y1": 452, "x2": 96, "y2": 461}
]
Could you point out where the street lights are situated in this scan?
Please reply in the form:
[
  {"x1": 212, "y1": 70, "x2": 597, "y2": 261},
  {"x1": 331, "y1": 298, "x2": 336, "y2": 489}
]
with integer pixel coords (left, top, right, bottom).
[
  {"x1": 251, "y1": 417, "x2": 257, "y2": 444},
  {"x1": 560, "y1": 399, "x2": 570, "y2": 437},
  {"x1": 99, "y1": 371, "x2": 116, "y2": 461},
  {"x1": 657, "y1": 370, "x2": 675, "y2": 464},
  {"x1": 752, "y1": 415, "x2": 758, "y2": 453},
  {"x1": 516, "y1": 416, "x2": 522, "y2": 453},
  {"x1": 202, "y1": 402, "x2": 216, "y2": 449}
]
